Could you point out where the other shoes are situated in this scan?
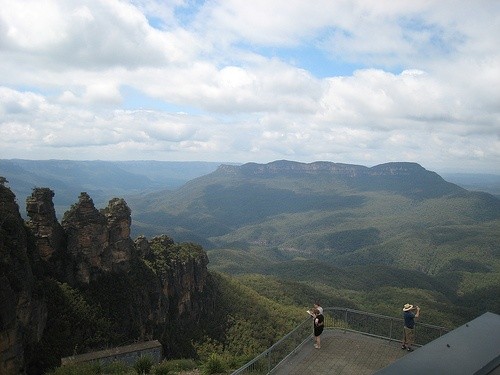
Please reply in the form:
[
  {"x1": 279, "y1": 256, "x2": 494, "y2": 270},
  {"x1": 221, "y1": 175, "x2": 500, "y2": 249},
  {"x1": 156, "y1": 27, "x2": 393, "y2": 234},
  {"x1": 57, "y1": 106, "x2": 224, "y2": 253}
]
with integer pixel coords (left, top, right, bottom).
[
  {"x1": 402, "y1": 345, "x2": 414, "y2": 352},
  {"x1": 314, "y1": 344, "x2": 320, "y2": 349}
]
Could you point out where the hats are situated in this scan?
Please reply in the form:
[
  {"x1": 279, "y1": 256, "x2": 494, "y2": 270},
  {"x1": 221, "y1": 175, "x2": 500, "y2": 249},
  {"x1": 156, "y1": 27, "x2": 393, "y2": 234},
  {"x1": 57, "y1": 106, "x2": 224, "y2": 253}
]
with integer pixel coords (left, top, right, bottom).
[{"x1": 403, "y1": 304, "x2": 413, "y2": 311}]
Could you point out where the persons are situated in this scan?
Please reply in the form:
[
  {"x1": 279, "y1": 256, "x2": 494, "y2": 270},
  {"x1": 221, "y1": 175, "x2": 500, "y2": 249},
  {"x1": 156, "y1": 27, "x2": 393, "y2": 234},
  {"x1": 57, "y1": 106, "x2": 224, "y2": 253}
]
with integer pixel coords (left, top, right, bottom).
[
  {"x1": 313, "y1": 309, "x2": 324, "y2": 349},
  {"x1": 401, "y1": 303, "x2": 420, "y2": 352},
  {"x1": 311, "y1": 302, "x2": 324, "y2": 342}
]
